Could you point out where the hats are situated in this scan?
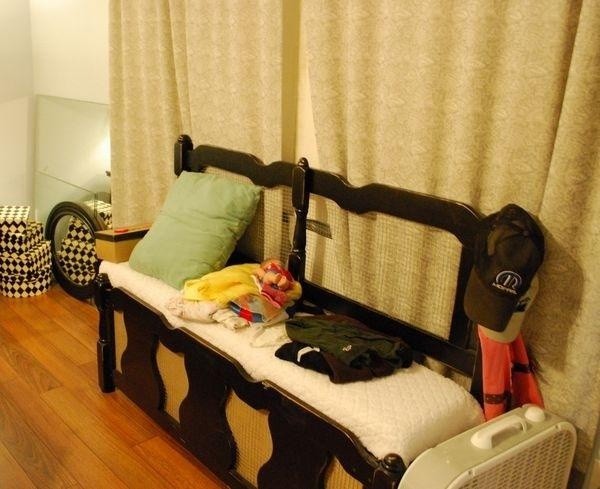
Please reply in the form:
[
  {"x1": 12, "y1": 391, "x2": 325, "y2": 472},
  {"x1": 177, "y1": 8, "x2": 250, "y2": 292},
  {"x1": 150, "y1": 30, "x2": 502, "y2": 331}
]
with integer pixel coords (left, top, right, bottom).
[{"x1": 464, "y1": 204, "x2": 544, "y2": 343}]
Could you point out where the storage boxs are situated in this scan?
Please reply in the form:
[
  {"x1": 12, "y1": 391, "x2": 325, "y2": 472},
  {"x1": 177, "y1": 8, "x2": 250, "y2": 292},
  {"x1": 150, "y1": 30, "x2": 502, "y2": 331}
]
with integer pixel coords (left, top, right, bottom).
[
  {"x1": 0, "y1": 199, "x2": 112, "y2": 297},
  {"x1": 95, "y1": 224, "x2": 151, "y2": 264}
]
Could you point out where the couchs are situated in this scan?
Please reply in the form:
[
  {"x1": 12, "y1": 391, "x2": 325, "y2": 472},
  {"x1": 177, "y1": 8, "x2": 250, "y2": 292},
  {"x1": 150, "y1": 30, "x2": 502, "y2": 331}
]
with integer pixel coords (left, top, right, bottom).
[{"x1": 96, "y1": 134, "x2": 484, "y2": 488}]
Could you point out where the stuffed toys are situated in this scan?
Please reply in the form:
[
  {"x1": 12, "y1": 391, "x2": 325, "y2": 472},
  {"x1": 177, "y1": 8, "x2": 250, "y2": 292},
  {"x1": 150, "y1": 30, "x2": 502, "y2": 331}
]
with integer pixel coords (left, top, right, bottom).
[{"x1": 257, "y1": 259, "x2": 294, "y2": 291}]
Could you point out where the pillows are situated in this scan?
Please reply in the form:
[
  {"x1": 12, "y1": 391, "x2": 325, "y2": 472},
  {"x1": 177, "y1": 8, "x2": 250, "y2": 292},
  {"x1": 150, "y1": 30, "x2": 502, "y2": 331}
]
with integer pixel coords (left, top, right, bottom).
[{"x1": 128, "y1": 171, "x2": 263, "y2": 291}]
